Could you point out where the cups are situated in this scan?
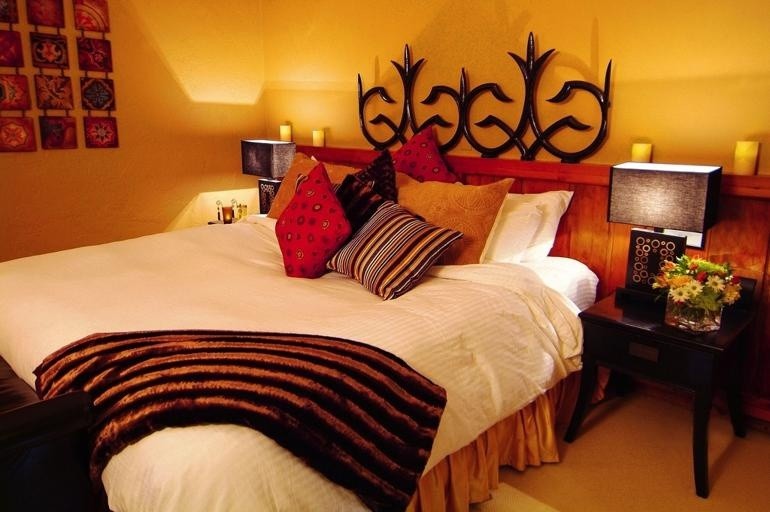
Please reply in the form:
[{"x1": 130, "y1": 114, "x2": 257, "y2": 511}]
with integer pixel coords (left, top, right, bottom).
[
  {"x1": 631, "y1": 144, "x2": 651, "y2": 163},
  {"x1": 221, "y1": 206, "x2": 234, "y2": 224},
  {"x1": 280, "y1": 124, "x2": 291, "y2": 142}
]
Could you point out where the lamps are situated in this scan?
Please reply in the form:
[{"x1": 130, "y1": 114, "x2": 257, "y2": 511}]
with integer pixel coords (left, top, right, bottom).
[
  {"x1": 607, "y1": 161, "x2": 717, "y2": 314},
  {"x1": 241, "y1": 136, "x2": 297, "y2": 217}
]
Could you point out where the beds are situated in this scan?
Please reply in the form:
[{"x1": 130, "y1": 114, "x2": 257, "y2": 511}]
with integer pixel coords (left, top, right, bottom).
[{"x1": 1, "y1": 148, "x2": 770, "y2": 512}]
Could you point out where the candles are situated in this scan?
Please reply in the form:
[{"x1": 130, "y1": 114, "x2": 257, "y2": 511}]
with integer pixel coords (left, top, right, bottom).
[
  {"x1": 279, "y1": 125, "x2": 291, "y2": 143},
  {"x1": 632, "y1": 142, "x2": 653, "y2": 163}
]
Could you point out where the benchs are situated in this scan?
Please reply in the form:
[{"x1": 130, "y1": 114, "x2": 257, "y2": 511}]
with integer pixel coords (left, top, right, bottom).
[{"x1": 1, "y1": 353, "x2": 109, "y2": 512}]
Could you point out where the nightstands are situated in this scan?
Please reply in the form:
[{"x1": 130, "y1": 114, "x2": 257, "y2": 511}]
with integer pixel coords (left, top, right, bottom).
[{"x1": 562, "y1": 275, "x2": 758, "y2": 498}]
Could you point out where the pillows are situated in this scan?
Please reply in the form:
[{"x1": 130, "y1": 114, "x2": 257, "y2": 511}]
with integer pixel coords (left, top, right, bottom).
[
  {"x1": 481, "y1": 186, "x2": 574, "y2": 266},
  {"x1": 326, "y1": 200, "x2": 465, "y2": 300},
  {"x1": 275, "y1": 163, "x2": 351, "y2": 279},
  {"x1": 394, "y1": 171, "x2": 516, "y2": 265},
  {"x1": 331, "y1": 149, "x2": 397, "y2": 233},
  {"x1": 392, "y1": 128, "x2": 460, "y2": 184},
  {"x1": 267, "y1": 153, "x2": 364, "y2": 217}
]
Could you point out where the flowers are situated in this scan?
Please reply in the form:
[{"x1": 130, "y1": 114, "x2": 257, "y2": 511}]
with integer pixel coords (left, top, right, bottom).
[{"x1": 647, "y1": 255, "x2": 741, "y2": 313}]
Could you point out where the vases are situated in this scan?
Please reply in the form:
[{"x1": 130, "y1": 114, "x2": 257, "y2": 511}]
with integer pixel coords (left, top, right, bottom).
[{"x1": 663, "y1": 293, "x2": 721, "y2": 333}]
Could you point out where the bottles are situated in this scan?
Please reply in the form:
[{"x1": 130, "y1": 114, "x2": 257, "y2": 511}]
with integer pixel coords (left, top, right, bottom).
[{"x1": 235, "y1": 203, "x2": 248, "y2": 223}]
